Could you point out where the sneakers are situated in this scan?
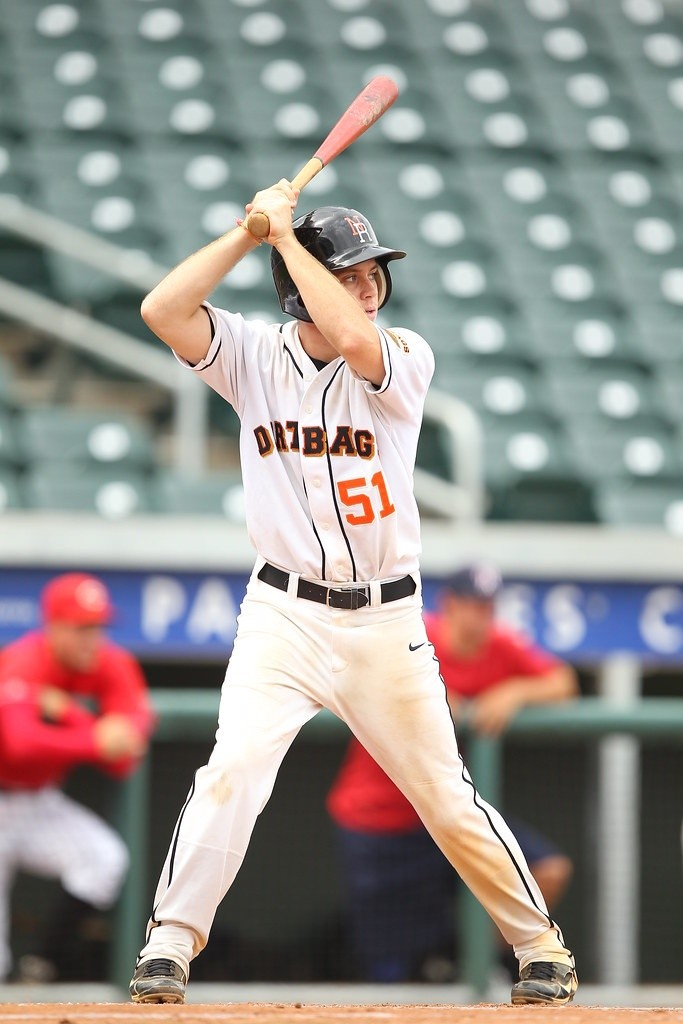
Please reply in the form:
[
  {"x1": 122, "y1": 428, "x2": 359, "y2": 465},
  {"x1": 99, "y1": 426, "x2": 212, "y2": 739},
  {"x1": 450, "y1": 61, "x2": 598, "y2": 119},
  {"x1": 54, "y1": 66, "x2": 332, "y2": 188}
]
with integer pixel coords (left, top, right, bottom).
[
  {"x1": 126, "y1": 956, "x2": 195, "y2": 1007},
  {"x1": 511, "y1": 959, "x2": 579, "y2": 1007}
]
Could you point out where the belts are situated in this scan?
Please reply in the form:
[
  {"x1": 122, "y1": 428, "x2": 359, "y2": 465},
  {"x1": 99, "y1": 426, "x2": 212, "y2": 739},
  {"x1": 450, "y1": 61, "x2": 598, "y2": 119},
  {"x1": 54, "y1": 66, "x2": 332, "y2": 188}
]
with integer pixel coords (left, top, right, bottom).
[{"x1": 252, "y1": 559, "x2": 422, "y2": 614}]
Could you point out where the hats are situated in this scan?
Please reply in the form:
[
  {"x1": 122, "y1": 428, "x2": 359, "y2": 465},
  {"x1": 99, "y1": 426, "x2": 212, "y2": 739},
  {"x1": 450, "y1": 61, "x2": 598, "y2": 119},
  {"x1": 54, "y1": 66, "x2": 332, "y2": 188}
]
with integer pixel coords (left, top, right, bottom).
[
  {"x1": 453, "y1": 568, "x2": 500, "y2": 614},
  {"x1": 38, "y1": 574, "x2": 119, "y2": 631}
]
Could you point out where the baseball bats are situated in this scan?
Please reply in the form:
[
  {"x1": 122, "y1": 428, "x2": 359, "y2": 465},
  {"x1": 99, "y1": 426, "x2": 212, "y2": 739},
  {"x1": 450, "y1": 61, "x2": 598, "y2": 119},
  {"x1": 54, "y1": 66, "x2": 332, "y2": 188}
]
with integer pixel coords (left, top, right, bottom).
[{"x1": 247, "y1": 75, "x2": 401, "y2": 236}]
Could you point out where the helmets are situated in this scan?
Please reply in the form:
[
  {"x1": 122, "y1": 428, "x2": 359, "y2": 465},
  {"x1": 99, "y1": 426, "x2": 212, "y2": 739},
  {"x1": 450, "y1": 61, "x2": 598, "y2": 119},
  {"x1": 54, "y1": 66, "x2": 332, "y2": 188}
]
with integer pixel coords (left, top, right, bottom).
[{"x1": 272, "y1": 206, "x2": 410, "y2": 291}]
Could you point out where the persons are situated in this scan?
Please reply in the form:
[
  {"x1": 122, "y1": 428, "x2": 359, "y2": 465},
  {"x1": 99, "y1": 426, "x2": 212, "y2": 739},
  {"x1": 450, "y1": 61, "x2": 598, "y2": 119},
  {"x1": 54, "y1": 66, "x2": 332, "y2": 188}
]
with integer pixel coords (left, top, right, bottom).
[{"x1": 130, "y1": 203, "x2": 580, "y2": 1006}]
